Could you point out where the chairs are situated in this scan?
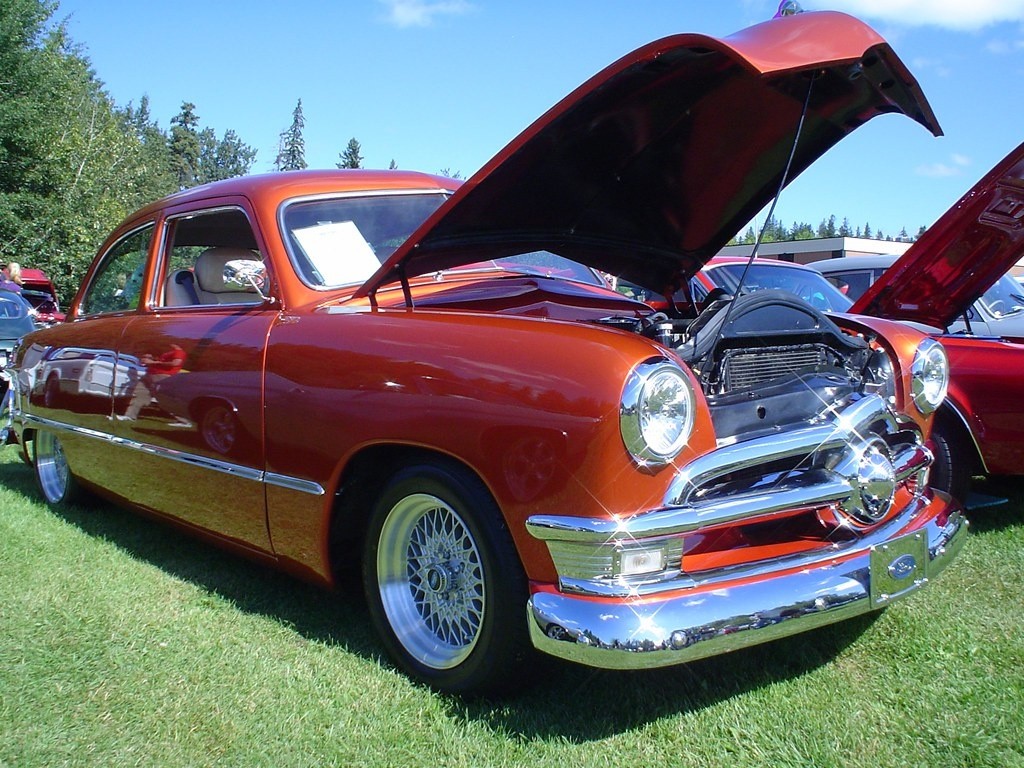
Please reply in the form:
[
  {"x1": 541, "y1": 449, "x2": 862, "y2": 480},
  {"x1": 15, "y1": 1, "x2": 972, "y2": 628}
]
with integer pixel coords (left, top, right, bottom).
[{"x1": 164, "y1": 247, "x2": 261, "y2": 306}]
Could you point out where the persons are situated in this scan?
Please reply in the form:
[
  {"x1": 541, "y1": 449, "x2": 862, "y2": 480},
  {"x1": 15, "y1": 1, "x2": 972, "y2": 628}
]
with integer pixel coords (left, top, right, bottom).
[
  {"x1": 118, "y1": 339, "x2": 194, "y2": 427},
  {"x1": 7, "y1": 263, "x2": 26, "y2": 286}
]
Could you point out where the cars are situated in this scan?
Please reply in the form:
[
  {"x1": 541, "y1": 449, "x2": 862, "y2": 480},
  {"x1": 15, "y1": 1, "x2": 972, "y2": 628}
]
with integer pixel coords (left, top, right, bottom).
[
  {"x1": 17, "y1": 289, "x2": 66, "y2": 332},
  {"x1": 1, "y1": 267, "x2": 60, "y2": 311},
  {"x1": 803, "y1": 255, "x2": 1024, "y2": 344},
  {"x1": 0, "y1": 289, "x2": 37, "y2": 395},
  {"x1": 603, "y1": 142, "x2": 1024, "y2": 502},
  {"x1": 9, "y1": 14, "x2": 971, "y2": 696}
]
